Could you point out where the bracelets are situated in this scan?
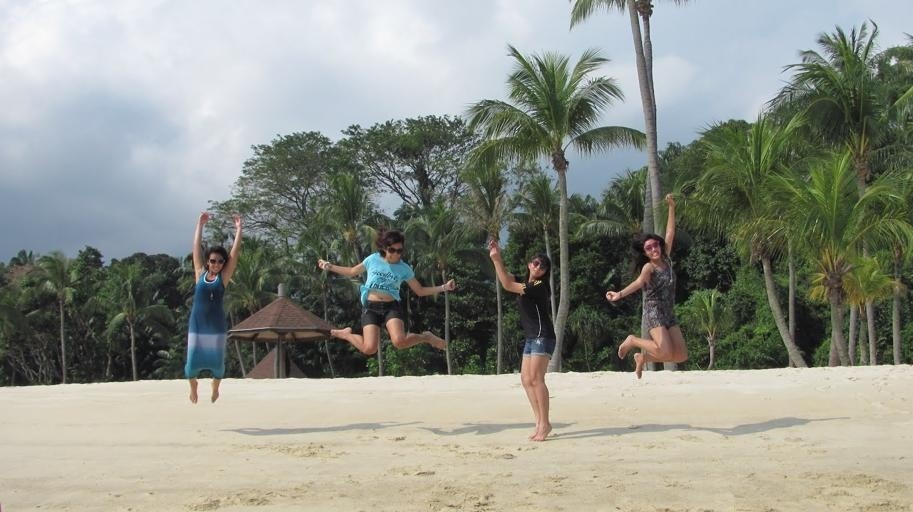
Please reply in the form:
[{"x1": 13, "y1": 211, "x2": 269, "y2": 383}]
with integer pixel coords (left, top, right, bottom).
[
  {"x1": 618, "y1": 291, "x2": 622, "y2": 299},
  {"x1": 442, "y1": 284, "x2": 446, "y2": 292}
]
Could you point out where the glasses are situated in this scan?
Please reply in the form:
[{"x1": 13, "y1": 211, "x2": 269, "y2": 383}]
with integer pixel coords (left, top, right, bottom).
[
  {"x1": 388, "y1": 248, "x2": 403, "y2": 254},
  {"x1": 645, "y1": 242, "x2": 658, "y2": 251},
  {"x1": 533, "y1": 261, "x2": 544, "y2": 269},
  {"x1": 210, "y1": 259, "x2": 225, "y2": 264}
]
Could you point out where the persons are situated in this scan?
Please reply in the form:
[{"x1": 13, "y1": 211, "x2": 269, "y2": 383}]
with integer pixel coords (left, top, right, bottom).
[
  {"x1": 484, "y1": 241, "x2": 557, "y2": 441},
  {"x1": 184, "y1": 210, "x2": 242, "y2": 403},
  {"x1": 317, "y1": 229, "x2": 455, "y2": 354},
  {"x1": 603, "y1": 193, "x2": 688, "y2": 378}
]
